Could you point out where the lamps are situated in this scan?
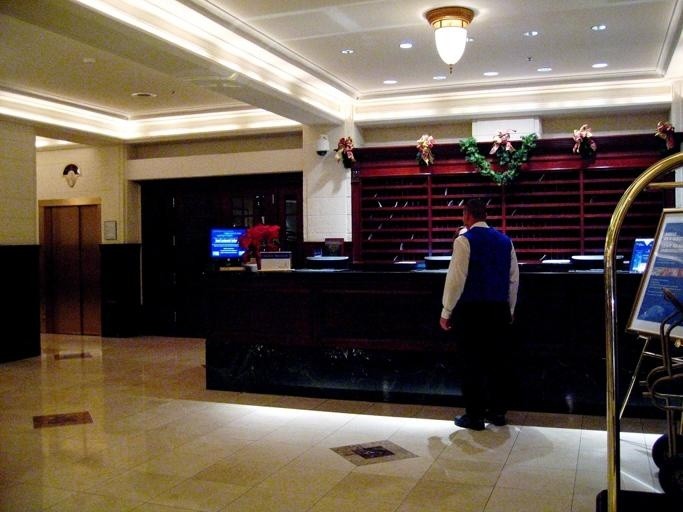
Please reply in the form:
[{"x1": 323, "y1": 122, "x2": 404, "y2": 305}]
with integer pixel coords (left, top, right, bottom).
[{"x1": 423, "y1": 6, "x2": 475, "y2": 76}]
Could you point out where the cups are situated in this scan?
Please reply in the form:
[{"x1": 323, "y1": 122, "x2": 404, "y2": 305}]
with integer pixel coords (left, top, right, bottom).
[{"x1": 313, "y1": 247, "x2": 322, "y2": 258}]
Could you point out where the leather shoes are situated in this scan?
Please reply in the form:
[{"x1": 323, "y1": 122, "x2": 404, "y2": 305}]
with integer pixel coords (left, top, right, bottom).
[
  {"x1": 453, "y1": 412, "x2": 486, "y2": 431},
  {"x1": 484, "y1": 411, "x2": 507, "y2": 426}
]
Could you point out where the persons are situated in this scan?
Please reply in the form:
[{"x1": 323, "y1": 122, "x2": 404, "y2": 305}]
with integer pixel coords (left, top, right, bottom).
[{"x1": 440, "y1": 197, "x2": 519, "y2": 431}]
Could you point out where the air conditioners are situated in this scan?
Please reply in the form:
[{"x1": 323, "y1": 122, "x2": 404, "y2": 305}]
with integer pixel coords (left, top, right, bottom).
[{"x1": 472, "y1": 116, "x2": 543, "y2": 144}]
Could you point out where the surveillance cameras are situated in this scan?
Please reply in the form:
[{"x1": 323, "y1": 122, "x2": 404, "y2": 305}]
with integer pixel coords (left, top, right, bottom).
[{"x1": 315, "y1": 134, "x2": 329, "y2": 156}]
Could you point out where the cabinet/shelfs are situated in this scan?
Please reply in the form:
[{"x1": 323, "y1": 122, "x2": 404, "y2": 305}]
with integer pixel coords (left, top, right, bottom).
[{"x1": 335, "y1": 127, "x2": 683, "y2": 265}]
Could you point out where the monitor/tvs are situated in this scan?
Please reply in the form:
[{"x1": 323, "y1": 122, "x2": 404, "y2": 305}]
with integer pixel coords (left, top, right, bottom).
[
  {"x1": 209, "y1": 227, "x2": 248, "y2": 267},
  {"x1": 571, "y1": 256, "x2": 623, "y2": 272},
  {"x1": 305, "y1": 256, "x2": 348, "y2": 270},
  {"x1": 425, "y1": 256, "x2": 452, "y2": 270}
]
